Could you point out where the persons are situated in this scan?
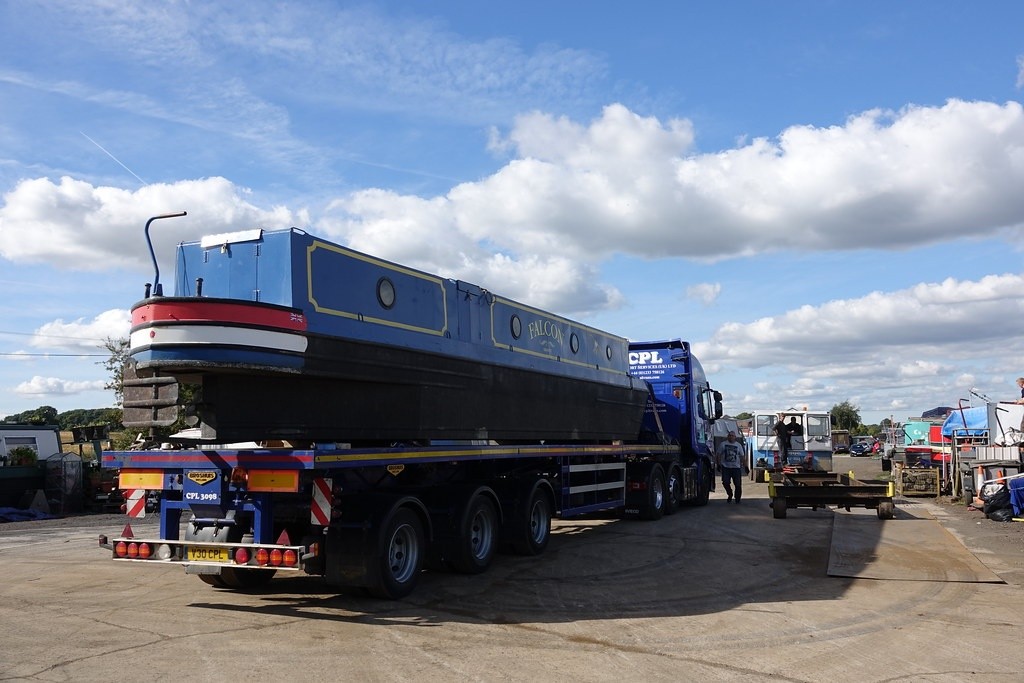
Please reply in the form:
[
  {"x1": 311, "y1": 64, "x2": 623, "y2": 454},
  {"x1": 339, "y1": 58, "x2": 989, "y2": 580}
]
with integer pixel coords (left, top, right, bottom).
[
  {"x1": 772, "y1": 413, "x2": 802, "y2": 468},
  {"x1": 1015, "y1": 377, "x2": 1024, "y2": 404},
  {"x1": 716, "y1": 431, "x2": 749, "y2": 505}
]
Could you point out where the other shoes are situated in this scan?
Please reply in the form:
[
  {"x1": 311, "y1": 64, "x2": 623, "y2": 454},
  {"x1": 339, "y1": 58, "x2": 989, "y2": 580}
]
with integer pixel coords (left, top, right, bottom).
[
  {"x1": 735, "y1": 497, "x2": 740, "y2": 503},
  {"x1": 727, "y1": 496, "x2": 732, "y2": 503}
]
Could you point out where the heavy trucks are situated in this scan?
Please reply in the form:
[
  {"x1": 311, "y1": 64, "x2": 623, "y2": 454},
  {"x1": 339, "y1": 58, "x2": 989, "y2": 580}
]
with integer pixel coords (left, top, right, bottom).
[
  {"x1": 743, "y1": 409, "x2": 832, "y2": 484},
  {"x1": 96, "y1": 212, "x2": 724, "y2": 605}
]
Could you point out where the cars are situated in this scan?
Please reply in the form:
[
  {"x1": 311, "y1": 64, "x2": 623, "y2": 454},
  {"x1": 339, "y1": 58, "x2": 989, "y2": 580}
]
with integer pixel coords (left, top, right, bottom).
[{"x1": 833, "y1": 441, "x2": 873, "y2": 457}]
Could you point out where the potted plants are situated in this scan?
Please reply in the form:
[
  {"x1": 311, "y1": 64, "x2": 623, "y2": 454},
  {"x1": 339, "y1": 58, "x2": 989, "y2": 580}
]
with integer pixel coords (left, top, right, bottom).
[{"x1": 5, "y1": 447, "x2": 38, "y2": 466}]
